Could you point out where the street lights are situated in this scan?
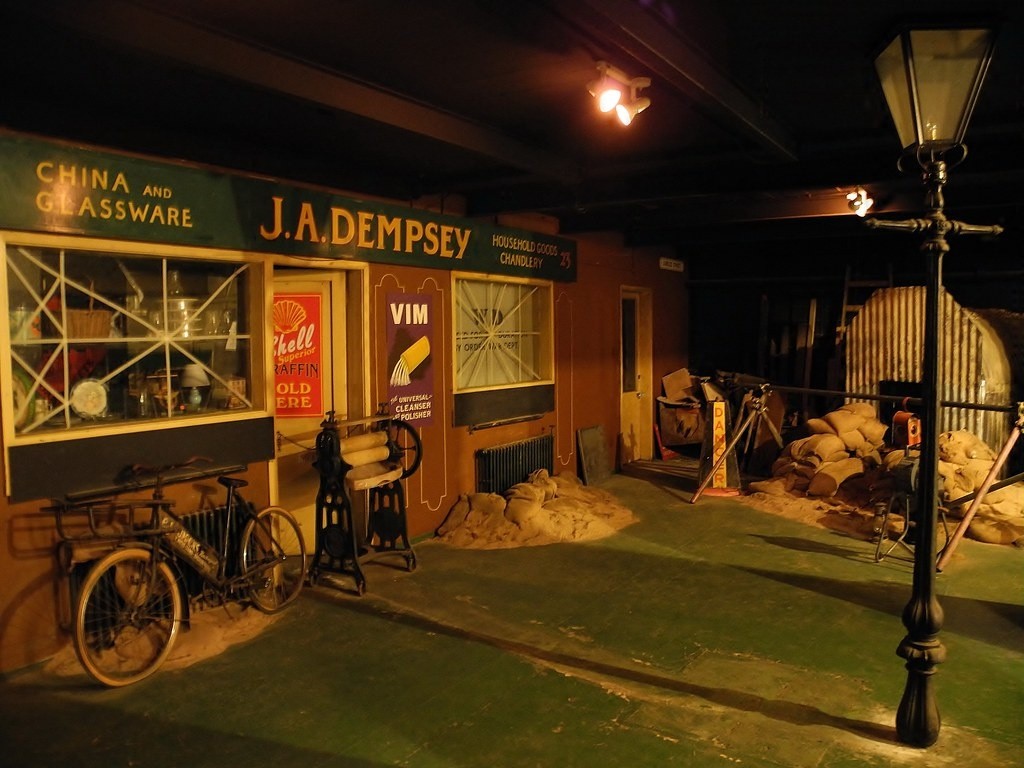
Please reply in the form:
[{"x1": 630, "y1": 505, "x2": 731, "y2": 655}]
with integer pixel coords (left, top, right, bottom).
[{"x1": 877, "y1": 4, "x2": 1005, "y2": 747}]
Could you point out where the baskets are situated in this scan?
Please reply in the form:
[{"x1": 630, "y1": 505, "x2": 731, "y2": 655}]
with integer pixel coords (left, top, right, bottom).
[{"x1": 46, "y1": 279, "x2": 113, "y2": 340}]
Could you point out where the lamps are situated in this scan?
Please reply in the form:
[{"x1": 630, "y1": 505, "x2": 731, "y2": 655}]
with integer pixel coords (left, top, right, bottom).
[
  {"x1": 181, "y1": 364, "x2": 210, "y2": 407},
  {"x1": 586, "y1": 61, "x2": 651, "y2": 126},
  {"x1": 846, "y1": 185, "x2": 874, "y2": 218}
]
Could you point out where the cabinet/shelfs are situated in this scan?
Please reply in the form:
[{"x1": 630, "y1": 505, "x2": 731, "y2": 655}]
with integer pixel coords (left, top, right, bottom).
[{"x1": 6, "y1": 247, "x2": 254, "y2": 436}]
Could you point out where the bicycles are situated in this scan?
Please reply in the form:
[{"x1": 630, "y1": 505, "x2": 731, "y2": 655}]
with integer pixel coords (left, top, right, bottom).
[{"x1": 73, "y1": 452, "x2": 308, "y2": 689}]
